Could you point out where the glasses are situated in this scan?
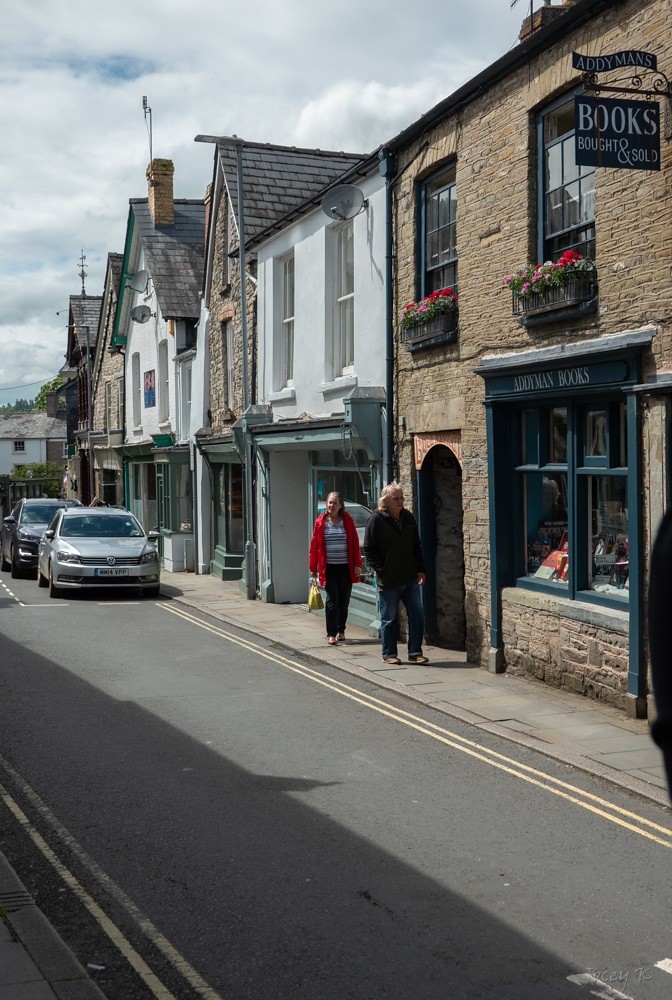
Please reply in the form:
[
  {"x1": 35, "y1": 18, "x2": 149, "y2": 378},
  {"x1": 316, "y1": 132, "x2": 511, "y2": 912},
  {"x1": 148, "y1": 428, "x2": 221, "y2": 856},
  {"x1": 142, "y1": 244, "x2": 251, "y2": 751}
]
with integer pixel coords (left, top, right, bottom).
[{"x1": 387, "y1": 496, "x2": 405, "y2": 501}]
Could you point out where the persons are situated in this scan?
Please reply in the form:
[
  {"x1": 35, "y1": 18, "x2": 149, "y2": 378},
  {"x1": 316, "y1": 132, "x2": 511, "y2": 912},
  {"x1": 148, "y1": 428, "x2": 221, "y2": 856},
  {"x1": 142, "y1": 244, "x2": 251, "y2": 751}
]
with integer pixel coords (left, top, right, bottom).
[
  {"x1": 364, "y1": 483, "x2": 431, "y2": 665},
  {"x1": 309, "y1": 492, "x2": 365, "y2": 646}
]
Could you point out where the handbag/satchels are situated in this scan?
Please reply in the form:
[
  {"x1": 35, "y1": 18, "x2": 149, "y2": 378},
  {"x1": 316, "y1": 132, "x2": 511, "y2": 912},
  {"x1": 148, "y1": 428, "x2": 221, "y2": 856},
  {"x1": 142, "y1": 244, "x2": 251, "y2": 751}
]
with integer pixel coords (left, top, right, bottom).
[{"x1": 307, "y1": 577, "x2": 324, "y2": 612}]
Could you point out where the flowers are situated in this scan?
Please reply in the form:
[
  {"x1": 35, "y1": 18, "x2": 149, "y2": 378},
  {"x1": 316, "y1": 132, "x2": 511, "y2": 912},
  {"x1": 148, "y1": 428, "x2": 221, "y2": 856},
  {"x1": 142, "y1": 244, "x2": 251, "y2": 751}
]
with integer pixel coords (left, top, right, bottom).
[
  {"x1": 399, "y1": 287, "x2": 458, "y2": 330},
  {"x1": 501, "y1": 249, "x2": 595, "y2": 301}
]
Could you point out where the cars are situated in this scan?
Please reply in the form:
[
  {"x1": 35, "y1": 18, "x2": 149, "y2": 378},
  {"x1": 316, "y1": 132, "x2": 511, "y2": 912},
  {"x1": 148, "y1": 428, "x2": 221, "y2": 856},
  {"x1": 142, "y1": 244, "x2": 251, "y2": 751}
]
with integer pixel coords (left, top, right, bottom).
[
  {"x1": 37, "y1": 504, "x2": 163, "y2": 599},
  {"x1": 317, "y1": 497, "x2": 379, "y2": 555},
  {"x1": 0, "y1": 496, "x2": 85, "y2": 578}
]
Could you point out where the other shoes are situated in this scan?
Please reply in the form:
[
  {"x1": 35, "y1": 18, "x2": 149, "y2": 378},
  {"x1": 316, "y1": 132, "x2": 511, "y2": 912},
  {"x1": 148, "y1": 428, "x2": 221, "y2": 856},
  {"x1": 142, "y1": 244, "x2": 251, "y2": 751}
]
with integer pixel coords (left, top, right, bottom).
[
  {"x1": 327, "y1": 636, "x2": 337, "y2": 644},
  {"x1": 337, "y1": 632, "x2": 346, "y2": 640},
  {"x1": 382, "y1": 657, "x2": 401, "y2": 664},
  {"x1": 408, "y1": 655, "x2": 429, "y2": 663}
]
